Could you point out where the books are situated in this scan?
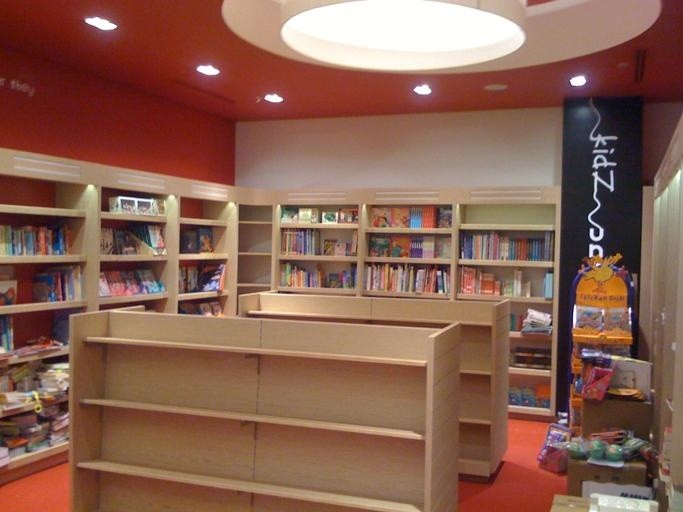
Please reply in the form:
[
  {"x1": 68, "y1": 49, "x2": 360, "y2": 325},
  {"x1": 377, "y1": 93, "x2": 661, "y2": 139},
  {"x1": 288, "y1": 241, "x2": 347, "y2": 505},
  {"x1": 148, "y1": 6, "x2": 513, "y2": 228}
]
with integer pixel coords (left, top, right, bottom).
[
  {"x1": 508, "y1": 302, "x2": 553, "y2": 410},
  {"x1": 96, "y1": 191, "x2": 229, "y2": 315},
  {"x1": 0, "y1": 192, "x2": 86, "y2": 474},
  {"x1": 274, "y1": 209, "x2": 556, "y2": 301}
]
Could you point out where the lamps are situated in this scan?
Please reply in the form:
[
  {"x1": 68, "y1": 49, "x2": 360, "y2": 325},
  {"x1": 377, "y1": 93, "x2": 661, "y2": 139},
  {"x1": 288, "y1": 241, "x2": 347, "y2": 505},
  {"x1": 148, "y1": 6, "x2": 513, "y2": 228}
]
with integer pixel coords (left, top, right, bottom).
[{"x1": 277, "y1": 0, "x2": 529, "y2": 73}]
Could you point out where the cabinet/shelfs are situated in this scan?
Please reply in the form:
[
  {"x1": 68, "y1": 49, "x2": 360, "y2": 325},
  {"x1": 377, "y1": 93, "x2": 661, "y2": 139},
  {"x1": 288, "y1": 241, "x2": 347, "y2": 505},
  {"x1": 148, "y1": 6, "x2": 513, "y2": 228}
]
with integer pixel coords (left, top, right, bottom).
[
  {"x1": 94, "y1": 163, "x2": 177, "y2": 315},
  {"x1": 239, "y1": 290, "x2": 513, "y2": 477},
  {"x1": 359, "y1": 185, "x2": 457, "y2": 295},
  {"x1": 454, "y1": 185, "x2": 563, "y2": 416},
  {"x1": 177, "y1": 178, "x2": 237, "y2": 317},
  {"x1": 235, "y1": 185, "x2": 275, "y2": 294},
  {"x1": 0, "y1": 145, "x2": 95, "y2": 479},
  {"x1": 67, "y1": 306, "x2": 464, "y2": 512},
  {"x1": 275, "y1": 188, "x2": 359, "y2": 289}
]
